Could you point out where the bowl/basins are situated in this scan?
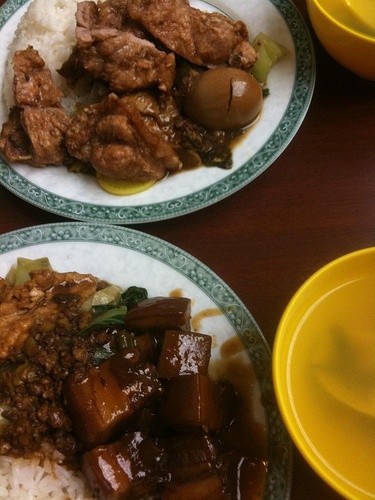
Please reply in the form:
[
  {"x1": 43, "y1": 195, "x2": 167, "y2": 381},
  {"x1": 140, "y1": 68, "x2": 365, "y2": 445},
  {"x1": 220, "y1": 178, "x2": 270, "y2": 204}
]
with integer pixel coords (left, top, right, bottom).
[
  {"x1": 307, "y1": 0, "x2": 375, "y2": 87},
  {"x1": 273, "y1": 241, "x2": 375, "y2": 500}
]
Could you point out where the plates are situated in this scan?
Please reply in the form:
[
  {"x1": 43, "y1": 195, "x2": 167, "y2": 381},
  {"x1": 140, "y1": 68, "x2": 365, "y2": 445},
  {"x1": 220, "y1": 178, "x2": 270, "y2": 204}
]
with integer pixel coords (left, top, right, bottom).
[
  {"x1": 1, "y1": 0, "x2": 316, "y2": 225},
  {"x1": 1, "y1": 222, "x2": 292, "y2": 500}
]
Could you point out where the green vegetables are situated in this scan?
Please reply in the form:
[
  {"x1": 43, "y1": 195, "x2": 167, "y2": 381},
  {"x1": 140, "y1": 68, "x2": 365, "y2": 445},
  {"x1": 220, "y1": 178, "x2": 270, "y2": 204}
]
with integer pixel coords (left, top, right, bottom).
[
  {"x1": 251, "y1": 32, "x2": 290, "y2": 82},
  {"x1": 66, "y1": 282, "x2": 147, "y2": 345}
]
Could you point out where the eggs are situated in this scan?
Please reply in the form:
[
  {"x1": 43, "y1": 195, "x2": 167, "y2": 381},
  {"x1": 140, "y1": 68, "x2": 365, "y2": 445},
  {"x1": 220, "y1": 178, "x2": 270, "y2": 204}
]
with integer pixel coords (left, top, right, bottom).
[{"x1": 185, "y1": 67, "x2": 263, "y2": 129}]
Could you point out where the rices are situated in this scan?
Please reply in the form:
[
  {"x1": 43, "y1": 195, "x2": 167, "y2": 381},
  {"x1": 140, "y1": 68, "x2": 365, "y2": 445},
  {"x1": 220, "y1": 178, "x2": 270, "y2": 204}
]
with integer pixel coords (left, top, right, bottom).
[
  {"x1": 2, "y1": 0, "x2": 97, "y2": 116},
  {"x1": 1, "y1": 451, "x2": 89, "y2": 499}
]
